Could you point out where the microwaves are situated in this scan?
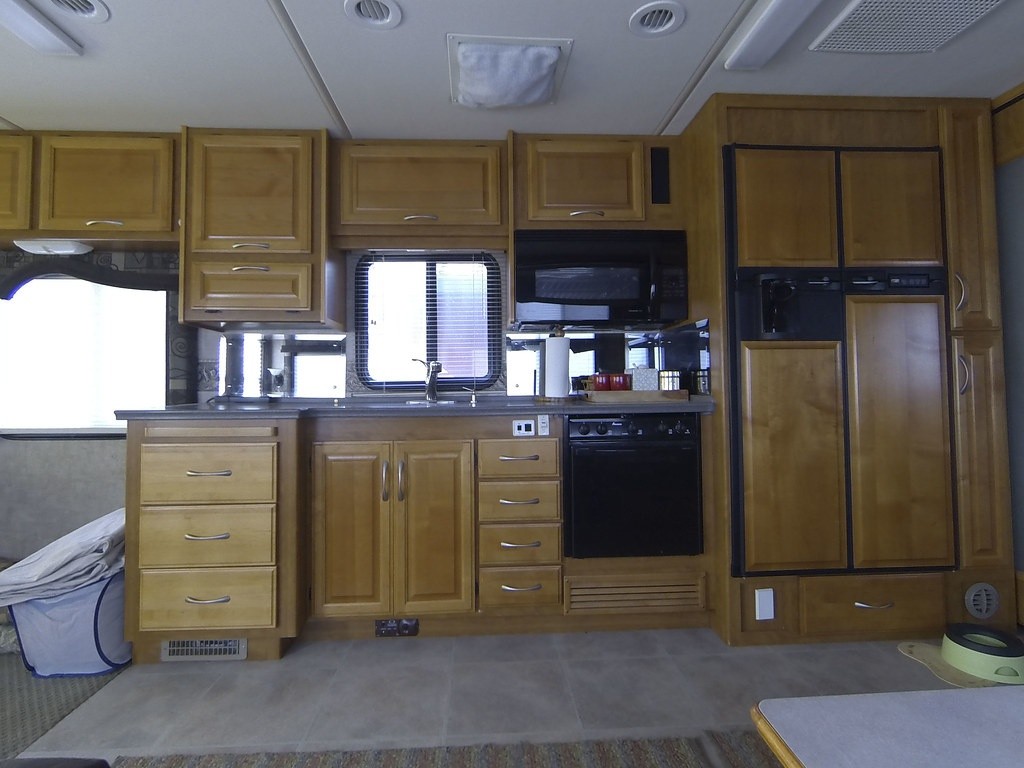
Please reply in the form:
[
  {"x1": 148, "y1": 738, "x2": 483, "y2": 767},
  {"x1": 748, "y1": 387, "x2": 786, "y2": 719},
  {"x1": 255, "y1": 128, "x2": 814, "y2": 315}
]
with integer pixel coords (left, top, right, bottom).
[{"x1": 513, "y1": 228, "x2": 688, "y2": 330}]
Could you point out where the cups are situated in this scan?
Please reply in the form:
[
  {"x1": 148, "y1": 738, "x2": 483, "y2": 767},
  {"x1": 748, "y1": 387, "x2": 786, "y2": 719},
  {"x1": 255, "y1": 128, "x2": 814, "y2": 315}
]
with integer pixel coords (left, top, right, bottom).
[
  {"x1": 609, "y1": 373, "x2": 632, "y2": 392},
  {"x1": 594, "y1": 373, "x2": 611, "y2": 392}
]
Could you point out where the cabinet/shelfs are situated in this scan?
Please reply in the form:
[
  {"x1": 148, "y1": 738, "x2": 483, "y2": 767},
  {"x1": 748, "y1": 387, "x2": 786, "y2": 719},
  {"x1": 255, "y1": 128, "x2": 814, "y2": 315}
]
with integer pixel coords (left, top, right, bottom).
[
  {"x1": 333, "y1": 139, "x2": 508, "y2": 252},
  {"x1": 0, "y1": 130, "x2": 180, "y2": 250},
  {"x1": 732, "y1": 147, "x2": 944, "y2": 268},
  {"x1": 736, "y1": 342, "x2": 851, "y2": 572},
  {"x1": 137, "y1": 427, "x2": 278, "y2": 628},
  {"x1": 526, "y1": 137, "x2": 645, "y2": 222},
  {"x1": 847, "y1": 293, "x2": 958, "y2": 570},
  {"x1": 479, "y1": 437, "x2": 561, "y2": 607},
  {"x1": 311, "y1": 440, "x2": 475, "y2": 616}
]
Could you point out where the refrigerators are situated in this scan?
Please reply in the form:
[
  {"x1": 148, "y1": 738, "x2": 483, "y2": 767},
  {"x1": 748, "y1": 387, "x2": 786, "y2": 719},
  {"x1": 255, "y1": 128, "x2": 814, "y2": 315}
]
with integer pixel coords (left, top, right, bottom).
[{"x1": 726, "y1": 146, "x2": 956, "y2": 577}]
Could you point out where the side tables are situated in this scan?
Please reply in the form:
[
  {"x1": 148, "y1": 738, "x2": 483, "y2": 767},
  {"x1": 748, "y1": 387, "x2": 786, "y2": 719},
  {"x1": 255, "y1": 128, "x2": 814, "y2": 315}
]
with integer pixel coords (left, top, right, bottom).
[{"x1": 179, "y1": 126, "x2": 345, "y2": 336}]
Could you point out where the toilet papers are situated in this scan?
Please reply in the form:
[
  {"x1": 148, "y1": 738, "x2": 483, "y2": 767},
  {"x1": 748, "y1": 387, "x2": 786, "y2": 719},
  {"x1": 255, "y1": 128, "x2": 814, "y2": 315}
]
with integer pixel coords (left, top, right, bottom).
[{"x1": 544, "y1": 336, "x2": 570, "y2": 398}]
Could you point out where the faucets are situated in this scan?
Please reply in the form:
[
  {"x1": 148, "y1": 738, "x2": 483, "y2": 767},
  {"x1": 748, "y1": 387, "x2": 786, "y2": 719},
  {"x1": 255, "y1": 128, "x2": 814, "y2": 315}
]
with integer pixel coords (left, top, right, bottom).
[{"x1": 412, "y1": 358, "x2": 442, "y2": 401}]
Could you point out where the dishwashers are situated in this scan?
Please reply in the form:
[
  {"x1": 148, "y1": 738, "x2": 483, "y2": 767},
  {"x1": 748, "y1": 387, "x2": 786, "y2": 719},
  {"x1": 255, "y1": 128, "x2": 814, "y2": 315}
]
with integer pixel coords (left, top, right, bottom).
[{"x1": 565, "y1": 414, "x2": 701, "y2": 557}]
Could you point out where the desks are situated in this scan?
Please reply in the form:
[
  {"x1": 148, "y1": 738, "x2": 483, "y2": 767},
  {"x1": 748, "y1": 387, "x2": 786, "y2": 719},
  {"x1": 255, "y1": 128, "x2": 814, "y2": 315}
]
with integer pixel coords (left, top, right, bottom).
[{"x1": 750, "y1": 684, "x2": 1024, "y2": 768}]
